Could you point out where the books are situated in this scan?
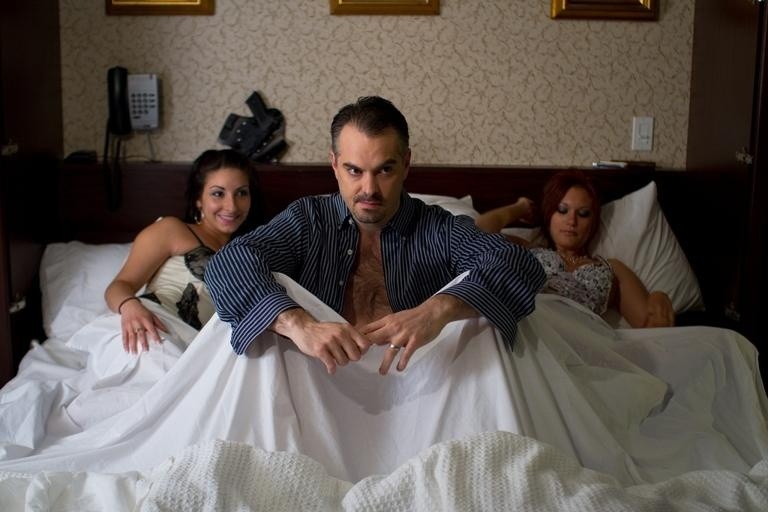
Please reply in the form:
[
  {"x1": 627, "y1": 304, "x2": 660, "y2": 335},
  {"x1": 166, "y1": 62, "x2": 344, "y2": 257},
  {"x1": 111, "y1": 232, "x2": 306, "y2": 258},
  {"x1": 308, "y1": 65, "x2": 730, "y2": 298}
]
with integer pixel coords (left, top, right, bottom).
[{"x1": 592, "y1": 161, "x2": 656, "y2": 170}]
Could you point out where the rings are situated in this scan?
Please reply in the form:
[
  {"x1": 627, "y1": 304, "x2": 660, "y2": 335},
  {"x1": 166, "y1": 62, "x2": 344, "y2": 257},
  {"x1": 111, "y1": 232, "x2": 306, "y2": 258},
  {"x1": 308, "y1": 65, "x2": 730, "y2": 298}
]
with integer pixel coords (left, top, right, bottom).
[
  {"x1": 133, "y1": 327, "x2": 145, "y2": 332},
  {"x1": 389, "y1": 344, "x2": 400, "y2": 351}
]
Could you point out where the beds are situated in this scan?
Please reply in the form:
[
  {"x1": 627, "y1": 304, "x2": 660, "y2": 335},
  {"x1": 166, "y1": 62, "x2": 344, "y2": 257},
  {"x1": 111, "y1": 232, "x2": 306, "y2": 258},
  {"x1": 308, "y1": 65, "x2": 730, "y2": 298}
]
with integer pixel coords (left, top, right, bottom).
[{"x1": 0, "y1": 161, "x2": 767, "y2": 512}]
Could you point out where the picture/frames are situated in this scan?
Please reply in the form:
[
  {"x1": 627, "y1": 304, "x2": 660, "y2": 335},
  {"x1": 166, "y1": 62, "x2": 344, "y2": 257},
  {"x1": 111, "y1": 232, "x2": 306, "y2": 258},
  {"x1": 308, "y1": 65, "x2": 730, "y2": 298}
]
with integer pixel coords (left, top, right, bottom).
[
  {"x1": 551, "y1": 0, "x2": 659, "y2": 22},
  {"x1": 106, "y1": 0, "x2": 214, "y2": 16},
  {"x1": 330, "y1": 0, "x2": 440, "y2": 15}
]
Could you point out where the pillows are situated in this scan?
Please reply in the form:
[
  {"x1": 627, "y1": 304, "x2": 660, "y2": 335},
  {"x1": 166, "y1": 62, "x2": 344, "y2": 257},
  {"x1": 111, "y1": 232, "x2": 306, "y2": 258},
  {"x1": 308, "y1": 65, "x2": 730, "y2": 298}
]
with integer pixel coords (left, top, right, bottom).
[
  {"x1": 39, "y1": 240, "x2": 149, "y2": 340},
  {"x1": 407, "y1": 192, "x2": 482, "y2": 228},
  {"x1": 500, "y1": 181, "x2": 707, "y2": 328}
]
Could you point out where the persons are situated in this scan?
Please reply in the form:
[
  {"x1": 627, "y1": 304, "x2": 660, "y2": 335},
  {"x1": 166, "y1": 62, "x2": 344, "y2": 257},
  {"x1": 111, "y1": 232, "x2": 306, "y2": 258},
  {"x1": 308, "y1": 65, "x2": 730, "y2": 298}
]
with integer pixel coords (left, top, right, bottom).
[
  {"x1": 474, "y1": 168, "x2": 675, "y2": 328},
  {"x1": 205, "y1": 96, "x2": 547, "y2": 375},
  {"x1": 105, "y1": 150, "x2": 264, "y2": 356}
]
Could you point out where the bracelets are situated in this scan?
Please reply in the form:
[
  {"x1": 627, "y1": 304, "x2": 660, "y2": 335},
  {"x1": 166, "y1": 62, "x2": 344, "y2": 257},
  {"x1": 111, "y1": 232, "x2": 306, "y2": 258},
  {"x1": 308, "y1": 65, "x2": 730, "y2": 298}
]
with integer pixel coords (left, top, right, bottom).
[{"x1": 118, "y1": 296, "x2": 143, "y2": 315}]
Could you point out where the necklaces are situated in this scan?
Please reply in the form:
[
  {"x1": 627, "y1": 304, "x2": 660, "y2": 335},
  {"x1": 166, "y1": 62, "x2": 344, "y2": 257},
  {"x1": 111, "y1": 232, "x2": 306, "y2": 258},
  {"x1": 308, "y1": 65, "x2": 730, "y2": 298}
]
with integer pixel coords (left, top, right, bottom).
[{"x1": 557, "y1": 250, "x2": 590, "y2": 265}]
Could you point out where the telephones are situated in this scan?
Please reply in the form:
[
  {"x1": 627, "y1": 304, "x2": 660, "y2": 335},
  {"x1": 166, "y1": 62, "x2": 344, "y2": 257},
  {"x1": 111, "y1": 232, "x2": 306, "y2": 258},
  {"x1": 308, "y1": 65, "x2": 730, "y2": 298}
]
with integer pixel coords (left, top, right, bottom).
[{"x1": 105, "y1": 66, "x2": 161, "y2": 135}]
